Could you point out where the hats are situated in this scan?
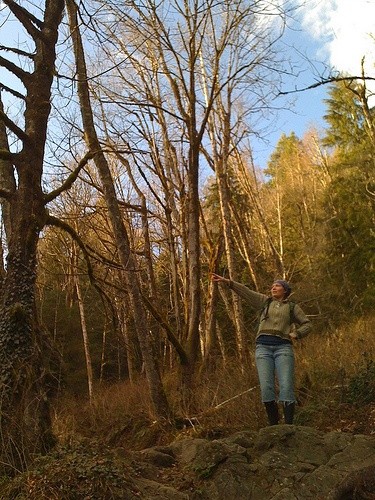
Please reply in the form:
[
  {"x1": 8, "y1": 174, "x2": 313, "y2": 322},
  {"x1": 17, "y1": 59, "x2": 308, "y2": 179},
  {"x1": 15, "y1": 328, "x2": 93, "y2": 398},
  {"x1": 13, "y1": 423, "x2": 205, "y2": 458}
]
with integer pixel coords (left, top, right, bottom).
[{"x1": 275, "y1": 280, "x2": 291, "y2": 300}]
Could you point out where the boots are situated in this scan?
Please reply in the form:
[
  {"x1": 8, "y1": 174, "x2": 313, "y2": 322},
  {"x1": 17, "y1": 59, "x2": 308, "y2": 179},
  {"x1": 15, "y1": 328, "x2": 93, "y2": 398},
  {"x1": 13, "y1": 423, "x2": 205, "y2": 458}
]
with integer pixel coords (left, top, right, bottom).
[
  {"x1": 283, "y1": 401, "x2": 294, "y2": 424},
  {"x1": 264, "y1": 400, "x2": 279, "y2": 425}
]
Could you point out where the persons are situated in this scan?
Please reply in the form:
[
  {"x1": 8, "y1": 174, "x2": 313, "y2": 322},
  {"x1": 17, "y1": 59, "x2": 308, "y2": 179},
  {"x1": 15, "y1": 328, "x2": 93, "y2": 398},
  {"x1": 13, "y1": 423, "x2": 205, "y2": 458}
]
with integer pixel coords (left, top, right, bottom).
[{"x1": 207, "y1": 271, "x2": 313, "y2": 426}]
